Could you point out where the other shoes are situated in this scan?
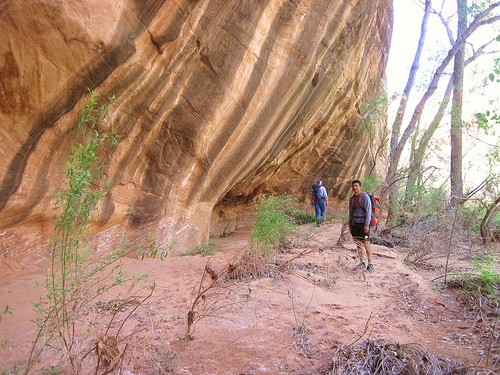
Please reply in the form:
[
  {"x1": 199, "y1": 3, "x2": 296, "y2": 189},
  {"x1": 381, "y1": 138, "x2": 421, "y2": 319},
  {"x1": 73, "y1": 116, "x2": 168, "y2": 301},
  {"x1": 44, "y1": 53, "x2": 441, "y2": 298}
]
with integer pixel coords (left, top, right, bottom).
[
  {"x1": 367, "y1": 264, "x2": 373, "y2": 270},
  {"x1": 353, "y1": 262, "x2": 366, "y2": 271}
]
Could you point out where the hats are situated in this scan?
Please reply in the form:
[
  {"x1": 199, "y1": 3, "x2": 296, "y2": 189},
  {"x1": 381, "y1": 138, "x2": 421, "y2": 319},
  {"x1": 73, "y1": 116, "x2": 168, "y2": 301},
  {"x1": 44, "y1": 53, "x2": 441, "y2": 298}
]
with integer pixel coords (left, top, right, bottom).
[{"x1": 317, "y1": 181, "x2": 322, "y2": 185}]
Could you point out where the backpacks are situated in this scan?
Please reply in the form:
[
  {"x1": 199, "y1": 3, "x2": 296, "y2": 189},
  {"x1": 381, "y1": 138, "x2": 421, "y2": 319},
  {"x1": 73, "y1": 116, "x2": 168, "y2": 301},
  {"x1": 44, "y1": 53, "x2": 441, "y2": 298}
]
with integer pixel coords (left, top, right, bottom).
[
  {"x1": 351, "y1": 192, "x2": 381, "y2": 226},
  {"x1": 312, "y1": 184, "x2": 323, "y2": 201}
]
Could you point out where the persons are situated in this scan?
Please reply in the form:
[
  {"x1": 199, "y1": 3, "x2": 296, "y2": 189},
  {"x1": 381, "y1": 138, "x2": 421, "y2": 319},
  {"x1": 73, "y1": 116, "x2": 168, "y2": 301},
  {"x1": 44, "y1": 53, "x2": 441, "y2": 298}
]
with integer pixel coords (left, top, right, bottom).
[
  {"x1": 312, "y1": 180, "x2": 329, "y2": 227},
  {"x1": 348, "y1": 180, "x2": 373, "y2": 271}
]
